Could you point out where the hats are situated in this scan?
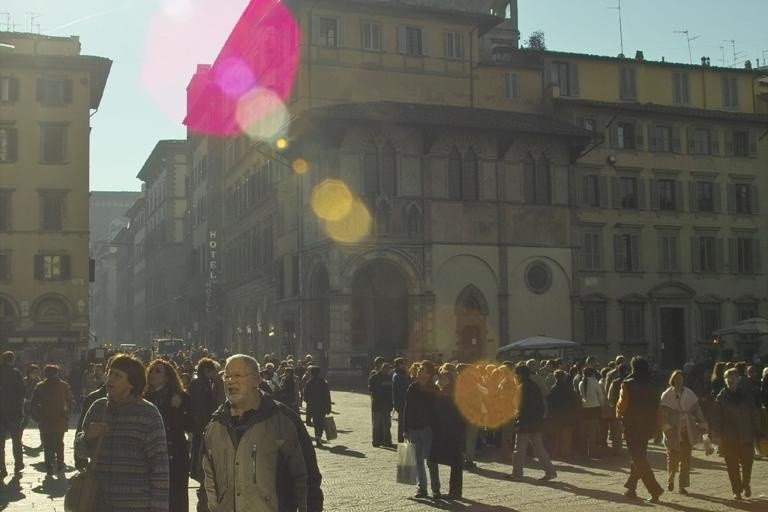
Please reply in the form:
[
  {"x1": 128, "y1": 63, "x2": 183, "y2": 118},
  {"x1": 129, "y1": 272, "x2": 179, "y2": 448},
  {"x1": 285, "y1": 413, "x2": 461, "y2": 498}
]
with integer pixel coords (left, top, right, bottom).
[{"x1": 394, "y1": 357, "x2": 406, "y2": 364}]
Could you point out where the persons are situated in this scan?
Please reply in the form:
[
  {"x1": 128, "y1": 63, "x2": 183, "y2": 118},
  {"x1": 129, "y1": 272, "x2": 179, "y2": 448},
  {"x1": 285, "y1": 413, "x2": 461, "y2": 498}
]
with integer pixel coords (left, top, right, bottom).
[
  {"x1": 74, "y1": 353, "x2": 198, "y2": 512},
  {"x1": 70, "y1": 345, "x2": 331, "y2": 475},
  {"x1": 1, "y1": 350, "x2": 76, "y2": 477},
  {"x1": 616, "y1": 354, "x2": 768, "y2": 503},
  {"x1": 367, "y1": 357, "x2": 631, "y2": 481},
  {"x1": 368, "y1": 352, "x2": 768, "y2": 502},
  {"x1": 203, "y1": 353, "x2": 324, "y2": 512}
]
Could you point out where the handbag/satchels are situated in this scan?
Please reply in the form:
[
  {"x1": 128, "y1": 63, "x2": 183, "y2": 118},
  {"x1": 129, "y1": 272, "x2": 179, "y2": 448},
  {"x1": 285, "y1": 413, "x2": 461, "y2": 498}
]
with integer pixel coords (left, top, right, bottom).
[
  {"x1": 395, "y1": 437, "x2": 419, "y2": 486},
  {"x1": 325, "y1": 413, "x2": 337, "y2": 439},
  {"x1": 64, "y1": 471, "x2": 100, "y2": 511}
]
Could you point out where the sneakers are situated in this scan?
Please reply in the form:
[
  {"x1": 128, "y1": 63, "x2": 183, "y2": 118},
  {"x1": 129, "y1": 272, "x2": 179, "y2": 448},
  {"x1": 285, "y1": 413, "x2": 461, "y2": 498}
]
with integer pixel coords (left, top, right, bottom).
[
  {"x1": 439, "y1": 493, "x2": 462, "y2": 500},
  {"x1": 743, "y1": 486, "x2": 752, "y2": 496},
  {"x1": 623, "y1": 489, "x2": 637, "y2": 497},
  {"x1": 503, "y1": 473, "x2": 521, "y2": 479},
  {"x1": 733, "y1": 492, "x2": 742, "y2": 498},
  {"x1": 538, "y1": 471, "x2": 557, "y2": 481},
  {"x1": 679, "y1": 487, "x2": 687, "y2": 494},
  {"x1": 667, "y1": 483, "x2": 673, "y2": 491},
  {"x1": 415, "y1": 489, "x2": 427, "y2": 497},
  {"x1": 647, "y1": 488, "x2": 663, "y2": 501},
  {"x1": 433, "y1": 491, "x2": 441, "y2": 499}
]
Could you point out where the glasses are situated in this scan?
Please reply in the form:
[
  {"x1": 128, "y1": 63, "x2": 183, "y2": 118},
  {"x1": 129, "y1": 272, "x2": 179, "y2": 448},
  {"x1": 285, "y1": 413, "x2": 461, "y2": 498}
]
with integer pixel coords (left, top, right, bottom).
[
  {"x1": 222, "y1": 373, "x2": 260, "y2": 382},
  {"x1": 416, "y1": 367, "x2": 429, "y2": 373}
]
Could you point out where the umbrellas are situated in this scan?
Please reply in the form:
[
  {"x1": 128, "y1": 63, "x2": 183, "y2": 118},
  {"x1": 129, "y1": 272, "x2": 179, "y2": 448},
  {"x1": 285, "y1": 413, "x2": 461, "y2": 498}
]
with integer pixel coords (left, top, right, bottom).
[{"x1": 712, "y1": 316, "x2": 768, "y2": 352}]
